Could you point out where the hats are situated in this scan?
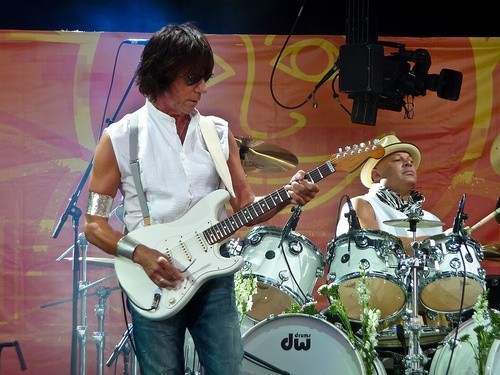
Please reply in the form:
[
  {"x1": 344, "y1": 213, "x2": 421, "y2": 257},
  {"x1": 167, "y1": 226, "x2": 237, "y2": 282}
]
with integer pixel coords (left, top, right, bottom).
[{"x1": 361, "y1": 135, "x2": 420, "y2": 188}]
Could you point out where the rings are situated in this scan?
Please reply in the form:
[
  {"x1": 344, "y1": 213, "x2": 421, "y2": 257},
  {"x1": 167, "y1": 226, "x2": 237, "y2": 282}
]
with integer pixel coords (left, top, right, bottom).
[{"x1": 159, "y1": 279, "x2": 164, "y2": 284}]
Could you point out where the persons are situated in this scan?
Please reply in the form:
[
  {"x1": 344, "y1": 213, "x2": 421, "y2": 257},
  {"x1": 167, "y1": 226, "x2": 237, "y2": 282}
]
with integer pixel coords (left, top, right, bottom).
[
  {"x1": 335, "y1": 135, "x2": 471, "y2": 258},
  {"x1": 84, "y1": 23, "x2": 319, "y2": 375}
]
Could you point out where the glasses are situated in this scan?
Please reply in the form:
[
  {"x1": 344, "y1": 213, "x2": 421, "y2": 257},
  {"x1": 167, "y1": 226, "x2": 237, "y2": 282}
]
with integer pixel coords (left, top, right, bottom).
[{"x1": 180, "y1": 73, "x2": 213, "y2": 86}]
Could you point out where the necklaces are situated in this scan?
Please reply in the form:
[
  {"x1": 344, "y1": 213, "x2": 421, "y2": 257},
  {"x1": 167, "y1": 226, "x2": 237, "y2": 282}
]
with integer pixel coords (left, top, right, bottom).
[{"x1": 179, "y1": 119, "x2": 187, "y2": 137}]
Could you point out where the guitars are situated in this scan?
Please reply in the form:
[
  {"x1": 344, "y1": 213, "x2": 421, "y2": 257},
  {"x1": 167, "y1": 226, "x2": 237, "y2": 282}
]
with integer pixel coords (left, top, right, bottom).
[{"x1": 113, "y1": 137, "x2": 386, "y2": 321}]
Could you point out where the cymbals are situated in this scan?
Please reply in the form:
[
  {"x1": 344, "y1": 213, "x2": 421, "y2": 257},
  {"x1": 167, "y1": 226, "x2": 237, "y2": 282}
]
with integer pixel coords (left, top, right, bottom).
[
  {"x1": 63, "y1": 256, "x2": 114, "y2": 266},
  {"x1": 382, "y1": 216, "x2": 445, "y2": 228},
  {"x1": 234, "y1": 135, "x2": 298, "y2": 173},
  {"x1": 482, "y1": 243, "x2": 500, "y2": 262}
]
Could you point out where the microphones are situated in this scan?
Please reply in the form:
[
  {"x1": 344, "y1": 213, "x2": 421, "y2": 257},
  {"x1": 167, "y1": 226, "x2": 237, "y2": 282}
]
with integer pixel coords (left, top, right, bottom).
[
  {"x1": 451, "y1": 200, "x2": 463, "y2": 235},
  {"x1": 123, "y1": 38, "x2": 148, "y2": 46},
  {"x1": 289, "y1": 208, "x2": 301, "y2": 231},
  {"x1": 345, "y1": 198, "x2": 361, "y2": 231}
]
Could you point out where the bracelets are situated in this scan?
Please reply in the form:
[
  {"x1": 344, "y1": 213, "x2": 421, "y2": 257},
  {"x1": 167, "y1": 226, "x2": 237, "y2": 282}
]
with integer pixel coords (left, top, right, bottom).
[{"x1": 116, "y1": 235, "x2": 141, "y2": 260}]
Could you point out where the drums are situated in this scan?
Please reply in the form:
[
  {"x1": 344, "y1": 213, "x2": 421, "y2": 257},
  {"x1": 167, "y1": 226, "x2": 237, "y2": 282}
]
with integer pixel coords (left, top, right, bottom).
[
  {"x1": 327, "y1": 227, "x2": 411, "y2": 324},
  {"x1": 415, "y1": 232, "x2": 486, "y2": 314},
  {"x1": 369, "y1": 310, "x2": 453, "y2": 346},
  {"x1": 428, "y1": 309, "x2": 499, "y2": 375},
  {"x1": 183, "y1": 310, "x2": 259, "y2": 375},
  {"x1": 233, "y1": 225, "x2": 325, "y2": 324},
  {"x1": 242, "y1": 312, "x2": 386, "y2": 375}
]
